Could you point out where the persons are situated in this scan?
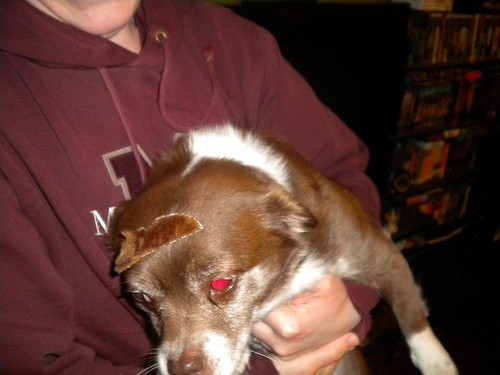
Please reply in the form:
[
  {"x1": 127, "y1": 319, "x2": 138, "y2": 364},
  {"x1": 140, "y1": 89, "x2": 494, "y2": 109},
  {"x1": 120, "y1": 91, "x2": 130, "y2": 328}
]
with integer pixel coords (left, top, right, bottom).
[{"x1": 0, "y1": 0, "x2": 383, "y2": 375}]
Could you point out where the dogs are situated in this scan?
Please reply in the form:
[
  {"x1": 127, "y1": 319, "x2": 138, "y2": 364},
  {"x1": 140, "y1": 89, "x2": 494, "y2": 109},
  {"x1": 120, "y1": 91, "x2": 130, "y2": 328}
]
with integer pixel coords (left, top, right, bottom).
[{"x1": 111, "y1": 118, "x2": 460, "y2": 375}]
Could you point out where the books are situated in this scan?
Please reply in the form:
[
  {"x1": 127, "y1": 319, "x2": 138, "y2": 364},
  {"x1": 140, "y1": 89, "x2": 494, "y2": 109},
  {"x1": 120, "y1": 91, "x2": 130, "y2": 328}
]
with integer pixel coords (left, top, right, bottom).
[{"x1": 380, "y1": 0, "x2": 499, "y2": 247}]
